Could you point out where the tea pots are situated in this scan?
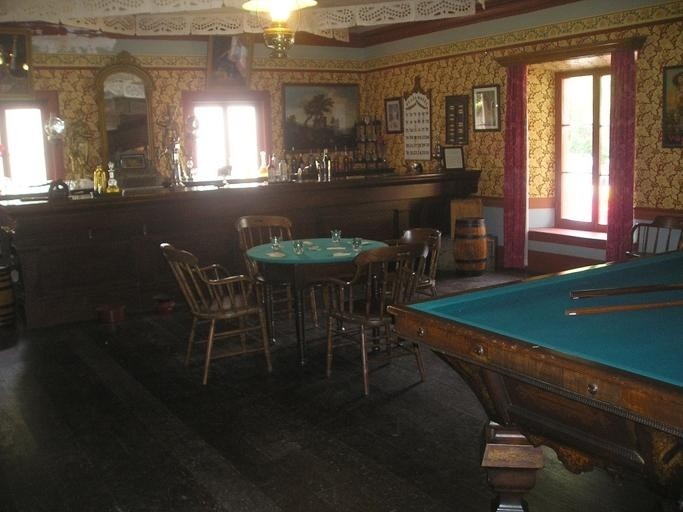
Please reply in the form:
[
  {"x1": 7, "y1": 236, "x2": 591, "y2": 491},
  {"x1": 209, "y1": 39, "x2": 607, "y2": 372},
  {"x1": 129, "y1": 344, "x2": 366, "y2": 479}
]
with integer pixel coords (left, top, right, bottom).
[
  {"x1": 42, "y1": 108, "x2": 66, "y2": 138},
  {"x1": 399, "y1": 159, "x2": 423, "y2": 175}
]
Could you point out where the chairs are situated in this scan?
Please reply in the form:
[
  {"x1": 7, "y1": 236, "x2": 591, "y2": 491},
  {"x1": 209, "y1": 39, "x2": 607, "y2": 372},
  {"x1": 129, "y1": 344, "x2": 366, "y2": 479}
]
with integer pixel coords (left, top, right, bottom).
[
  {"x1": 233, "y1": 214, "x2": 319, "y2": 345},
  {"x1": 0, "y1": 223, "x2": 27, "y2": 342},
  {"x1": 322, "y1": 245, "x2": 429, "y2": 395},
  {"x1": 158, "y1": 242, "x2": 273, "y2": 384},
  {"x1": 626, "y1": 222, "x2": 683, "y2": 261},
  {"x1": 369, "y1": 226, "x2": 443, "y2": 308}
]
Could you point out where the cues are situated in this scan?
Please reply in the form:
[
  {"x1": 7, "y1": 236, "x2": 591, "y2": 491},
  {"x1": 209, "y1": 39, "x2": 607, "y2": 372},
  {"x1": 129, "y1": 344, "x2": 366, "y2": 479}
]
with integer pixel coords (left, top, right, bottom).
[
  {"x1": 565, "y1": 301, "x2": 683, "y2": 317},
  {"x1": 570, "y1": 284, "x2": 683, "y2": 298}
]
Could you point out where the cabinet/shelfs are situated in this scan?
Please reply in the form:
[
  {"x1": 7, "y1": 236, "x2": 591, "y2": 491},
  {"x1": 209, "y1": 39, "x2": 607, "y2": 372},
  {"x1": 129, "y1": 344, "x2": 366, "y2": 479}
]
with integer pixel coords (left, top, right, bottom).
[{"x1": 348, "y1": 111, "x2": 388, "y2": 174}]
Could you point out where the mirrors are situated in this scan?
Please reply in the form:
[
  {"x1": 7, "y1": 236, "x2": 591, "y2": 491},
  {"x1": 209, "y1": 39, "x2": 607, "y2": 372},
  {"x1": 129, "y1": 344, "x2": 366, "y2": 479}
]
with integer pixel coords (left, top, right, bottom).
[{"x1": 92, "y1": 50, "x2": 153, "y2": 175}]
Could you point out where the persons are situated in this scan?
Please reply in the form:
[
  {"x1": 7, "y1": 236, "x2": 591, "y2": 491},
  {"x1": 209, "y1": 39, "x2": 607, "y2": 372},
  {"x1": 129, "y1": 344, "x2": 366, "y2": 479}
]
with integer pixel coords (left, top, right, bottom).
[{"x1": 388, "y1": 108, "x2": 400, "y2": 131}]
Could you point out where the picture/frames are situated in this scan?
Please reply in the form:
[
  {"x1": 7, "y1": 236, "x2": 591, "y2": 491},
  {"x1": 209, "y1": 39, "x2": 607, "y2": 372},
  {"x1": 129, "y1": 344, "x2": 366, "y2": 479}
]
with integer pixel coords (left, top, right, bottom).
[
  {"x1": 470, "y1": 84, "x2": 502, "y2": 134},
  {"x1": 281, "y1": 81, "x2": 361, "y2": 153},
  {"x1": 0, "y1": 26, "x2": 36, "y2": 102},
  {"x1": 205, "y1": 32, "x2": 251, "y2": 92},
  {"x1": 119, "y1": 153, "x2": 146, "y2": 171},
  {"x1": 383, "y1": 97, "x2": 404, "y2": 134},
  {"x1": 660, "y1": 65, "x2": 682, "y2": 149}
]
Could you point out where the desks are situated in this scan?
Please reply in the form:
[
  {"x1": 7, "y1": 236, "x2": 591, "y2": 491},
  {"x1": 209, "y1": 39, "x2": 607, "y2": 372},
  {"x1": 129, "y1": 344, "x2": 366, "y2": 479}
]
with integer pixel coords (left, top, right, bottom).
[
  {"x1": 383, "y1": 249, "x2": 683, "y2": 512},
  {"x1": 244, "y1": 237, "x2": 389, "y2": 367}
]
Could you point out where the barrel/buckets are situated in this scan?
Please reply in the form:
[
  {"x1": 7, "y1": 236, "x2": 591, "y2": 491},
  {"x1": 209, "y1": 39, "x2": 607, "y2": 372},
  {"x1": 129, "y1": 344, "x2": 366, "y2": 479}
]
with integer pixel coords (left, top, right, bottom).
[
  {"x1": 0, "y1": 265, "x2": 17, "y2": 350},
  {"x1": 451, "y1": 216, "x2": 489, "y2": 277}
]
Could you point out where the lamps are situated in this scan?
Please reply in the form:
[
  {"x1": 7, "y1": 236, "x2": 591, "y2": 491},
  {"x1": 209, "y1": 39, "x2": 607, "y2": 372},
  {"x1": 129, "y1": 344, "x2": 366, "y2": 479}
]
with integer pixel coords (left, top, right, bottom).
[{"x1": 239, "y1": 0, "x2": 318, "y2": 65}]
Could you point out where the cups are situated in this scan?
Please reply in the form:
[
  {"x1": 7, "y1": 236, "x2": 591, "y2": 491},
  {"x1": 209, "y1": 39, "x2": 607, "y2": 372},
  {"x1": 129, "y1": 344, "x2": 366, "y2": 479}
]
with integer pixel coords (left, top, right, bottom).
[
  {"x1": 291, "y1": 240, "x2": 303, "y2": 256},
  {"x1": 330, "y1": 230, "x2": 341, "y2": 243},
  {"x1": 351, "y1": 237, "x2": 362, "y2": 253},
  {"x1": 269, "y1": 236, "x2": 280, "y2": 251}
]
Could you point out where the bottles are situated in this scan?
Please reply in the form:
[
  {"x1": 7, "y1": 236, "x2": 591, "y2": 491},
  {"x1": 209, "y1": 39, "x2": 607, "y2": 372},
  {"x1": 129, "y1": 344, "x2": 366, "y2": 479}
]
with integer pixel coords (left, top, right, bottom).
[
  {"x1": 258, "y1": 143, "x2": 386, "y2": 183},
  {"x1": 352, "y1": 114, "x2": 384, "y2": 138},
  {"x1": 431, "y1": 136, "x2": 442, "y2": 166},
  {"x1": 92, "y1": 161, "x2": 118, "y2": 193}
]
